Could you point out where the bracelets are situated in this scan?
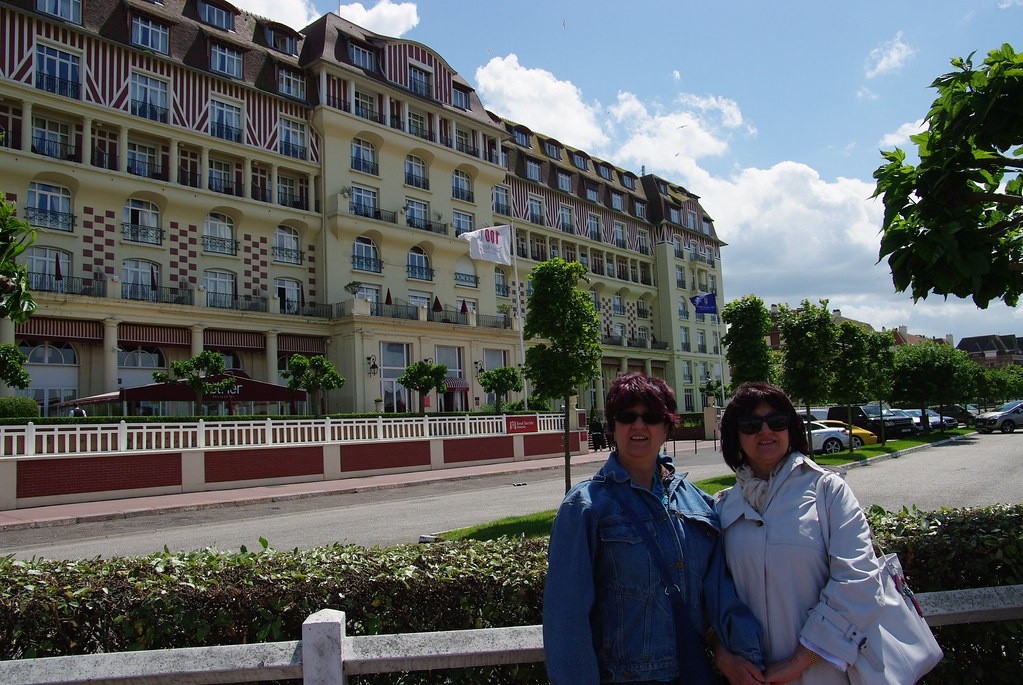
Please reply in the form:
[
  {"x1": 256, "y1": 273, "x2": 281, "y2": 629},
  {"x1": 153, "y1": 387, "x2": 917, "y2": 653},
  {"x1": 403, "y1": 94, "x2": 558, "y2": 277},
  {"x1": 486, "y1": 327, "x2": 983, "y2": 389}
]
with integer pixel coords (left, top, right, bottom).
[
  {"x1": 809, "y1": 650, "x2": 818, "y2": 664},
  {"x1": 705, "y1": 646, "x2": 720, "y2": 673}
]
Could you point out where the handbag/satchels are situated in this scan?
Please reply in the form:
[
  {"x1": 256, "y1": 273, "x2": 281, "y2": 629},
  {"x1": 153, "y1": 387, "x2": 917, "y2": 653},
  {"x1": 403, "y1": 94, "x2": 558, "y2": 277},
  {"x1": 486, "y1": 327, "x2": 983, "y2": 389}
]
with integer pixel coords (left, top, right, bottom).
[
  {"x1": 816, "y1": 471, "x2": 944, "y2": 684},
  {"x1": 677, "y1": 607, "x2": 718, "y2": 684}
]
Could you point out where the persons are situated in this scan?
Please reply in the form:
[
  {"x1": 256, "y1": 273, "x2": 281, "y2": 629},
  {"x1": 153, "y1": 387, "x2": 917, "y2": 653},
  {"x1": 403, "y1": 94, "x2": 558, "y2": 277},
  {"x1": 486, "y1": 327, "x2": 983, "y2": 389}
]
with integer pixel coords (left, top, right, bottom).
[
  {"x1": 605, "y1": 419, "x2": 614, "y2": 451},
  {"x1": 712, "y1": 380, "x2": 885, "y2": 685},
  {"x1": 538, "y1": 364, "x2": 763, "y2": 685},
  {"x1": 588, "y1": 417, "x2": 603, "y2": 452},
  {"x1": 69, "y1": 402, "x2": 86, "y2": 417}
]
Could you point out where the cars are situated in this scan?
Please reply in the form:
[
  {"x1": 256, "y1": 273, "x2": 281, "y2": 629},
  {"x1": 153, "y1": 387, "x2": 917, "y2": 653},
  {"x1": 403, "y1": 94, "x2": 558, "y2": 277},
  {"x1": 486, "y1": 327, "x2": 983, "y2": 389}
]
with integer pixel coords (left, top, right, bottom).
[
  {"x1": 928, "y1": 406, "x2": 978, "y2": 426},
  {"x1": 802, "y1": 421, "x2": 850, "y2": 455},
  {"x1": 974, "y1": 400, "x2": 1023, "y2": 433},
  {"x1": 815, "y1": 420, "x2": 878, "y2": 448},
  {"x1": 890, "y1": 409, "x2": 958, "y2": 432}
]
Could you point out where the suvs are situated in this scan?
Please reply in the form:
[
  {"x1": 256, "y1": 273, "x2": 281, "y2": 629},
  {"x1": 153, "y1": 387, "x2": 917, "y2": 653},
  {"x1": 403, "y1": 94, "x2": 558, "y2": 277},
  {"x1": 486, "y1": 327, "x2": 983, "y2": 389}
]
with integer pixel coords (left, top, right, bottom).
[{"x1": 827, "y1": 404, "x2": 916, "y2": 443}]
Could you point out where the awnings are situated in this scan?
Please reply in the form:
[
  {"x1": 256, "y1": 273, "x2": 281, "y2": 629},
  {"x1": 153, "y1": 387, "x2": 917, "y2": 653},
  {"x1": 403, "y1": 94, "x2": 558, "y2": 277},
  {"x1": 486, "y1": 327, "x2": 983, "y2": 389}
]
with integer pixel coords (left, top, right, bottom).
[{"x1": 445, "y1": 377, "x2": 469, "y2": 392}]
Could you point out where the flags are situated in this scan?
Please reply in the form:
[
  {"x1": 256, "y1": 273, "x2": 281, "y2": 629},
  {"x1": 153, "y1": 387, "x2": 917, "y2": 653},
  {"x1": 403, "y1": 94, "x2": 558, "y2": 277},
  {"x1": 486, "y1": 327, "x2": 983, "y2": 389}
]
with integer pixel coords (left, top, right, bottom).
[
  {"x1": 458, "y1": 224, "x2": 512, "y2": 266},
  {"x1": 689, "y1": 293, "x2": 718, "y2": 314}
]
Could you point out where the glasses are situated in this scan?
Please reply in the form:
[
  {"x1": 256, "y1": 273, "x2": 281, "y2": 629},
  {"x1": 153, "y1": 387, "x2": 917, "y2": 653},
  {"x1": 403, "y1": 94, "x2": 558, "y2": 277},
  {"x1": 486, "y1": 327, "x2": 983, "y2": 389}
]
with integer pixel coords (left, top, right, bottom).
[
  {"x1": 733, "y1": 411, "x2": 792, "y2": 434},
  {"x1": 609, "y1": 409, "x2": 668, "y2": 424}
]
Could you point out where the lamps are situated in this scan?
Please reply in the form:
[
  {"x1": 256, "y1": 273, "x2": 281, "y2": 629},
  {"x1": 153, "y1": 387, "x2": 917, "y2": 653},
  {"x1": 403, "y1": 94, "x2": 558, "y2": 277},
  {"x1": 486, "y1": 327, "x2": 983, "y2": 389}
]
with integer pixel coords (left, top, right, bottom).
[
  {"x1": 367, "y1": 354, "x2": 379, "y2": 377},
  {"x1": 473, "y1": 360, "x2": 485, "y2": 382},
  {"x1": 423, "y1": 358, "x2": 435, "y2": 366}
]
